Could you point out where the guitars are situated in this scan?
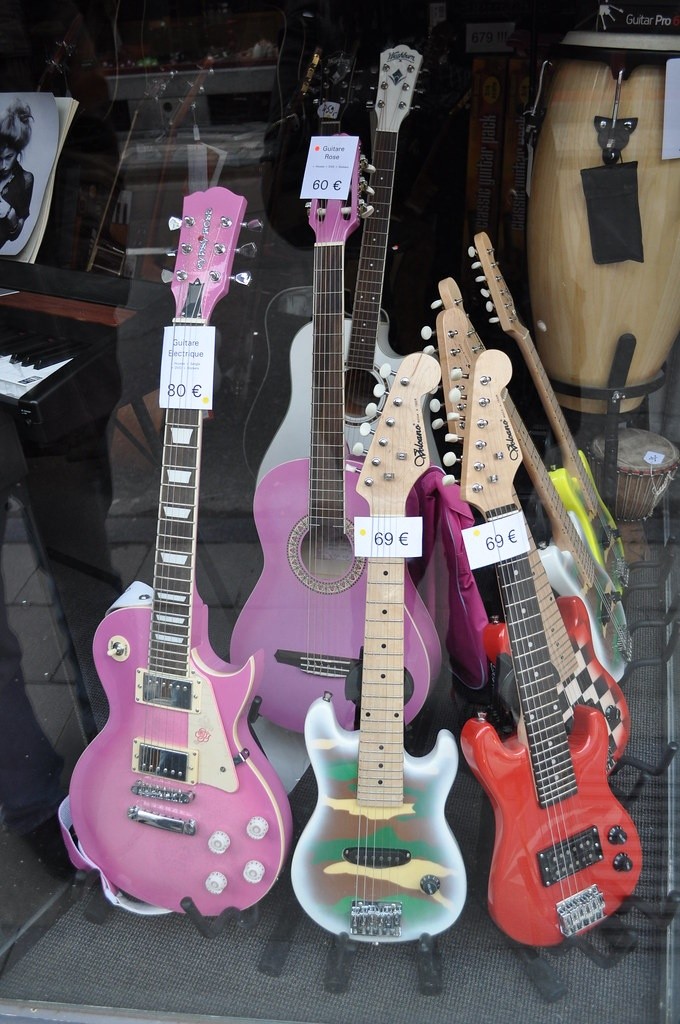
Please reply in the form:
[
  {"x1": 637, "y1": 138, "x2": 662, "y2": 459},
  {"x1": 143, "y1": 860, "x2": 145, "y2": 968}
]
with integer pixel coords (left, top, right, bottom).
[
  {"x1": 64, "y1": 187, "x2": 293, "y2": 920},
  {"x1": 224, "y1": 41, "x2": 449, "y2": 738},
  {"x1": 421, "y1": 231, "x2": 644, "y2": 945},
  {"x1": 290, "y1": 351, "x2": 469, "y2": 947},
  {"x1": 78, "y1": 52, "x2": 215, "y2": 288}
]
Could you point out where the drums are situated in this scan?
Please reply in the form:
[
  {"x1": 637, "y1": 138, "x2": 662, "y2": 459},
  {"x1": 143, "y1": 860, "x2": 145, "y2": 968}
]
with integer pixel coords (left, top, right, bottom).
[
  {"x1": 521, "y1": 29, "x2": 680, "y2": 418},
  {"x1": 587, "y1": 426, "x2": 680, "y2": 524}
]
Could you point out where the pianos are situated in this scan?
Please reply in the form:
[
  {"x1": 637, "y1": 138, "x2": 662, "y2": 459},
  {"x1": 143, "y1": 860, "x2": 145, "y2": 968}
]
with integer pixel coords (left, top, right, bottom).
[{"x1": 0, "y1": 256, "x2": 174, "y2": 496}]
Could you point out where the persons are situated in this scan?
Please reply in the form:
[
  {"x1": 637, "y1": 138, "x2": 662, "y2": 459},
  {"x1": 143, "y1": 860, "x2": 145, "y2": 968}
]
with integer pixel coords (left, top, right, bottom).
[{"x1": 1, "y1": 1, "x2": 130, "y2": 887}]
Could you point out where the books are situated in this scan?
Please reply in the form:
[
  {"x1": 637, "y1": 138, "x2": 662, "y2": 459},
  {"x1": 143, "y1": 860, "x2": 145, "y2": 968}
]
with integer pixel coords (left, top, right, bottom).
[
  {"x1": 0, "y1": 288, "x2": 20, "y2": 297},
  {"x1": 0, "y1": 91, "x2": 80, "y2": 264}
]
